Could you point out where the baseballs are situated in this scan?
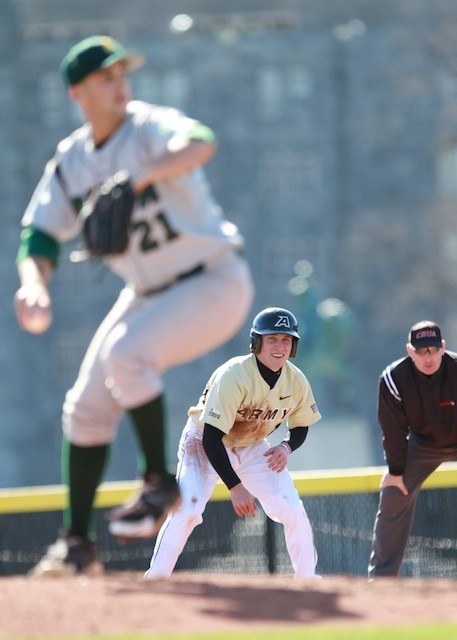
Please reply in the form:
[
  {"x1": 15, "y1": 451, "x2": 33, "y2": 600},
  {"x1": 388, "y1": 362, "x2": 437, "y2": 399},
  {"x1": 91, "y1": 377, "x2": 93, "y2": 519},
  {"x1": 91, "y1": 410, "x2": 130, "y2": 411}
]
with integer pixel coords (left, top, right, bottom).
[{"x1": 17, "y1": 305, "x2": 52, "y2": 334}]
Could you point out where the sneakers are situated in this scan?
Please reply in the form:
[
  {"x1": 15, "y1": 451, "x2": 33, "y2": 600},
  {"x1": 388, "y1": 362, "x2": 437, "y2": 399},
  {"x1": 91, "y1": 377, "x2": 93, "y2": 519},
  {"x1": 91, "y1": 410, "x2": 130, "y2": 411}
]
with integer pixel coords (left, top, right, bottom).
[
  {"x1": 108, "y1": 473, "x2": 182, "y2": 538},
  {"x1": 32, "y1": 537, "x2": 102, "y2": 577}
]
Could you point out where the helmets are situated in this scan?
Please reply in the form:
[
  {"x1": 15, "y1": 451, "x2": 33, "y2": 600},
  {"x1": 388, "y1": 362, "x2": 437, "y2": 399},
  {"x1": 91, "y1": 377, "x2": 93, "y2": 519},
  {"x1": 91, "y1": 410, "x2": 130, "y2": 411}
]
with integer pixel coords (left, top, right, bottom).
[{"x1": 249, "y1": 307, "x2": 300, "y2": 357}]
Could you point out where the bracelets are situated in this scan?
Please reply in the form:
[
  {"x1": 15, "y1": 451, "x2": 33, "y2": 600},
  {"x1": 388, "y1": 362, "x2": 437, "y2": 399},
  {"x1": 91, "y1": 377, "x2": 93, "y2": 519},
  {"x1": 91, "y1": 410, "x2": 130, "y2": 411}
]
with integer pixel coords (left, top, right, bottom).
[{"x1": 277, "y1": 443, "x2": 289, "y2": 455}]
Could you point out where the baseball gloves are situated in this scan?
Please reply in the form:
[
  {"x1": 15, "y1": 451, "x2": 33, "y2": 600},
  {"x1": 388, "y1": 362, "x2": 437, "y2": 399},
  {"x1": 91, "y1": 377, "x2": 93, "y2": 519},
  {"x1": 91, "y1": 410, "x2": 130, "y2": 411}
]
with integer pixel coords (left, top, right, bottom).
[{"x1": 79, "y1": 169, "x2": 133, "y2": 255}]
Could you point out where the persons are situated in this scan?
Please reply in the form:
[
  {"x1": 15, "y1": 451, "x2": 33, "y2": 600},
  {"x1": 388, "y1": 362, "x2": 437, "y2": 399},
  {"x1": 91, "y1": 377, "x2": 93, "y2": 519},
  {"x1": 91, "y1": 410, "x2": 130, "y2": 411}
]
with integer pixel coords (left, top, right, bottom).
[
  {"x1": 364, "y1": 318, "x2": 456, "y2": 589},
  {"x1": 14, "y1": 33, "x2": 253, "y2": 579},
  {"x1": 143, "y1": 307, "x2": 330, "y2": 583}
]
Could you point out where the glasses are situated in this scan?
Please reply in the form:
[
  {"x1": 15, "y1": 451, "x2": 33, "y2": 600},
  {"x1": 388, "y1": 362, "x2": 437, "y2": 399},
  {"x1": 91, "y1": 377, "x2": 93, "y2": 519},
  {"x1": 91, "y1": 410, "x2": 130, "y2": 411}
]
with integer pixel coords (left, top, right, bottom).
[{"x1": 415, "y1": 346, "x2": 440, "y2": 355}]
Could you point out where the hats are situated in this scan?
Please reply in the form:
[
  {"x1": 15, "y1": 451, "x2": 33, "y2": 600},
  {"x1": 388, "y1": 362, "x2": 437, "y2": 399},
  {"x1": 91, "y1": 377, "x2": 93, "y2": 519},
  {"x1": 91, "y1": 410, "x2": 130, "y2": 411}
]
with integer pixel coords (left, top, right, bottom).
[
  {"x1": 409, "y1": 320, "x2": 442, "y2": 348},
  {"x1": 58, "y1": 36, "x2": 143, "y2": 86}
]
